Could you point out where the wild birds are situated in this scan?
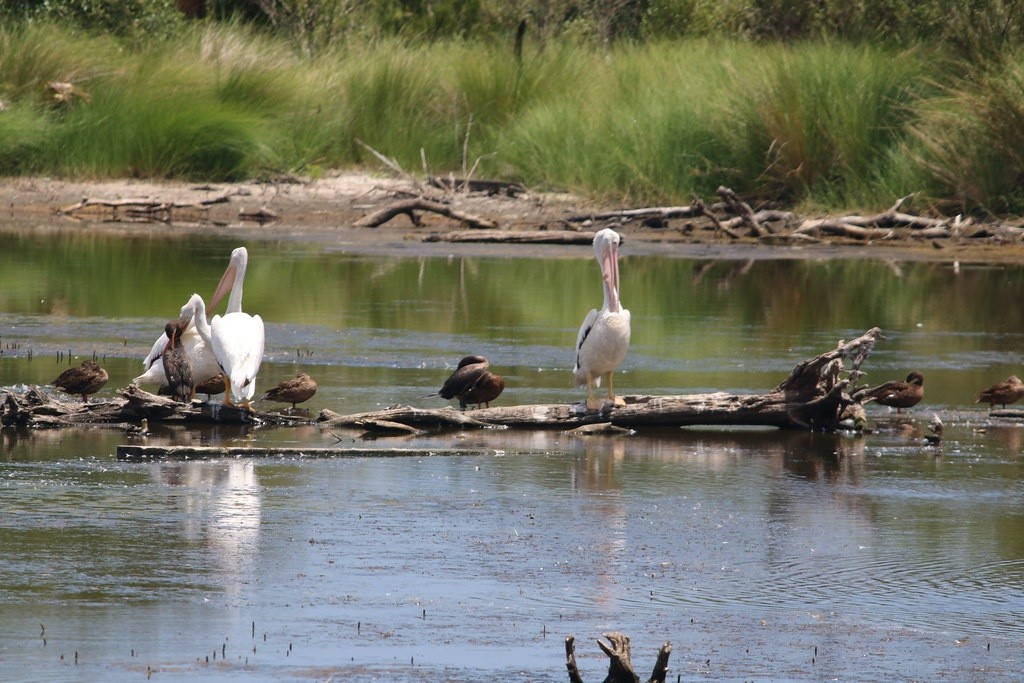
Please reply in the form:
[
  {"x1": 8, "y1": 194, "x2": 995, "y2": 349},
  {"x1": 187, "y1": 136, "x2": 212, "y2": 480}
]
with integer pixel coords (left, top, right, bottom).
[
  {"x1": 865, "y1": 369, "x2": 925, "y2": 415},
  {"x1": 438, "y1": 354, "x2": 505, "y2": 410},
  {"x1": 975, "y1": 374, "x2": 1024, "y2": 411},
  {"x1": 51, "y1": 359, "x2": 109, "y2": 404},
  {"x1": 131, "y1": 246, "x2": 266, "y2": 413},
  {"x1": 259, "y1": 370, "x2": 318, "y2": 410},
  {"x1": 572, "y1": 228, "x2": 632, "y2": 411}
]
